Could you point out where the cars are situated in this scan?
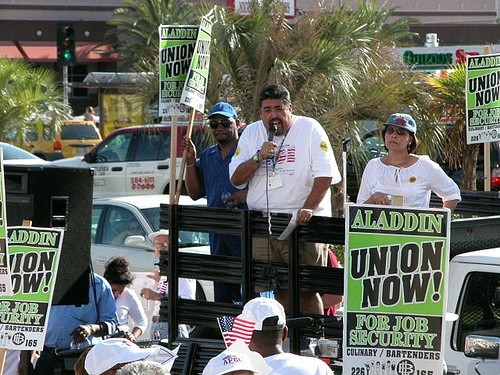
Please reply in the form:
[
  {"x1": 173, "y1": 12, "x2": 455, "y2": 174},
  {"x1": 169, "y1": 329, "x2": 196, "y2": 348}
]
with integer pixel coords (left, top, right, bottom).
[
  {"x1": 341, "y1": 128, "x2": 384, "y2": 202},
  {"x1": 0, "y1": 141, "x2": 48, "y2": 165},
  {"x1": 86, "y1": 194, "x2": 211, "y2": 302},
  {"x1": 441, "y1": 158, "x2": 500, "y2": 190},
  {"x1": 5, "y1": 121, "x2": 104, "y2": 162}
]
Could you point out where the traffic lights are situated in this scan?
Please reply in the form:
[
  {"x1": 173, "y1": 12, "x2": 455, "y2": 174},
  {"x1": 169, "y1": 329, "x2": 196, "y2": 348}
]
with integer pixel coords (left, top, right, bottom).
[{"x1": 56, "y1": 21, "x2": 76, "y2": 66}]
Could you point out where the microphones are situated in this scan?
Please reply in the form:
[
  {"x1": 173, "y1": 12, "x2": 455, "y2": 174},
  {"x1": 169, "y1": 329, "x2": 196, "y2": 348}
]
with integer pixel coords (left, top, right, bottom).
[{"x1": 268, "y1": 124, "x2": 278, "y2": 142}]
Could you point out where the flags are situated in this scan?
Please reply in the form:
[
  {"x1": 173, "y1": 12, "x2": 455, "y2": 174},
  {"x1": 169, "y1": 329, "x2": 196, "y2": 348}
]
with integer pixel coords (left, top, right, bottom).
[{"x1": 218, "y1": 316, "x2": 255, "y2": 348}]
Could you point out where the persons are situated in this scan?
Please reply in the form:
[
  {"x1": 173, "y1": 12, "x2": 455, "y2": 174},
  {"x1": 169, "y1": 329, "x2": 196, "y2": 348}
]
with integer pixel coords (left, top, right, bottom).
[
  {"x1": 241, "y1": 296, "x2": 335, "y2": 375},
  {"x1": 84, "y1": 106, "x2": 96, "y2": 122},
  {"x1": 316, "y1": 249, "x2": 343, "y2": 316},
  {"x1": 355, "y1": 114, "x2": 462, "y2": 216},
  {"x1": 0, "y1": 230, "x2": 197, "y2": 375},
  {"x1": 127, "y1": 219, "x2": 146, "y2": 238},
  {"x1": 227, "y1": 86, "x2": 343, "y2": 313},
  {"x1": 202, "y1": 348, "x2": 276, "y2": 375},
  {"x1": 182, "y1": 102, "x2": 249, "y2": 303}
]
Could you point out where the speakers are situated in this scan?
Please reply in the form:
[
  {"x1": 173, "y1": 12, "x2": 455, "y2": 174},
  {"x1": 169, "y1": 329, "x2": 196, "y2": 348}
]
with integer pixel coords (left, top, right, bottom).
[{"x1": 3, "y1": 164, "x2": 95, "y2": 304}]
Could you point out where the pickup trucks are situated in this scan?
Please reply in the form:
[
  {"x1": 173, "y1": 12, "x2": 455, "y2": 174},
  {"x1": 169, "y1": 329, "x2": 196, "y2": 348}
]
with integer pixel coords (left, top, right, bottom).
[{"x1": 52, "y1": 122, "x2": 246, "y2": 197}]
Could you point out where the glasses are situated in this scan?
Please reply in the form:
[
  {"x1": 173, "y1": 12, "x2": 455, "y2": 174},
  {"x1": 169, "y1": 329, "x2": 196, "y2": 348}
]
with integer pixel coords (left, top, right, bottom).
[
  {"x1": 209, "y1": 119, "x2": 237, "y2": 129},
  {"x1": 127, "y1": 226, "x2": 141, "y2": 231},
  {"x1": 153, "y1": 241, "x2": 169, "y2": 248},
  {"x1": 385, "y1": 127, "x2": 411, "y2": 136}
]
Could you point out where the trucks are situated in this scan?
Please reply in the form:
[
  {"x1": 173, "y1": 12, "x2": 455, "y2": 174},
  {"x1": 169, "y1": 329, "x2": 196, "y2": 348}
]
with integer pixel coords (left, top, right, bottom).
[{"x1": 154, "y1": 188, "x2": 499, "y2": 375}]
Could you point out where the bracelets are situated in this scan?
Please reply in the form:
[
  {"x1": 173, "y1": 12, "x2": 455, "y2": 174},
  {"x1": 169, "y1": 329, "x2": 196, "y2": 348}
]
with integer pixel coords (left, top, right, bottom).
[
  {"x1": 253, "y1": 152, "x2": 262, "y2": 164},
  {"x1": 185, "y1": 163, "x2": 196, "y2": 167}
]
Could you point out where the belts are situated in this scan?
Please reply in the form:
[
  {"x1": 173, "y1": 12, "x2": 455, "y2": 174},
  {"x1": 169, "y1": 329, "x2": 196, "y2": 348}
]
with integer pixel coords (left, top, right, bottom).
[
  {"x1": 152, "y1": 316, "x2": 161, "y2": 322},
  {"x1": 43, "y1": 345, "x2": 69, "y2": 356}
]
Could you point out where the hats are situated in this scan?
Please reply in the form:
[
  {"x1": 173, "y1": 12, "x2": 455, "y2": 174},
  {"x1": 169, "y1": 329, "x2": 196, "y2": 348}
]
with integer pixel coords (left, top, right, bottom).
[
  {"x1": 383, "y1": 113, "x2": 417, "y2": 133},
  {"x1": 206, "y1": 102, "x2": 239, "y2": 120},
  {"x1": 148, "y1": 228, "x2": 182, "y2": 242},
  {"x1": 84, "y1": 337, "x2": 160, "y2": 374},
  {"x1": 240, "y1": 296, "x2": 286, "y2": 331}
]
